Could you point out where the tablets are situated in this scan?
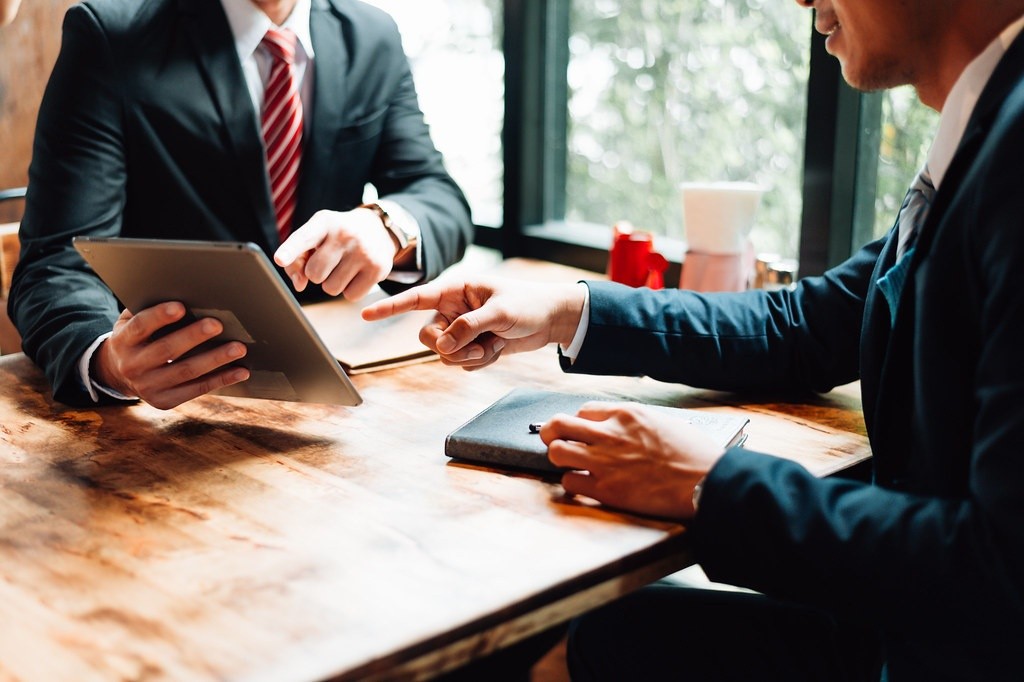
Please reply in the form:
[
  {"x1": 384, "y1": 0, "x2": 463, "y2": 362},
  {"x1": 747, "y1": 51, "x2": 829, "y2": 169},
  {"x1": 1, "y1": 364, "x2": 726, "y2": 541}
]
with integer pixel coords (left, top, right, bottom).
[{"x1": 72, "y1": 235, "x2": 363, "y2": 407}]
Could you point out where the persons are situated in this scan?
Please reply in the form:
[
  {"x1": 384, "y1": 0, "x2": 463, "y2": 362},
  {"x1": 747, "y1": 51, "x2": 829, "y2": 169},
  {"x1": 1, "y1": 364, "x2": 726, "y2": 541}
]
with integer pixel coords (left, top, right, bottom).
[
  {"x1": 357, "y1": 1, "x2": 1022, "y2": 682},
  {"x1": 5, "y1": 0, "x2": 473, "y2": 411}
]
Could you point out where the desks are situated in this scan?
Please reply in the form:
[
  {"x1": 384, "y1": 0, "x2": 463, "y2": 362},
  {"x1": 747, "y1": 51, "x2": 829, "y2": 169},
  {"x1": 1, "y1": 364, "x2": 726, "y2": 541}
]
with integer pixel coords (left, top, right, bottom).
[{"x1": 0, "y1": 257, "x2": 874, "y2": 682}]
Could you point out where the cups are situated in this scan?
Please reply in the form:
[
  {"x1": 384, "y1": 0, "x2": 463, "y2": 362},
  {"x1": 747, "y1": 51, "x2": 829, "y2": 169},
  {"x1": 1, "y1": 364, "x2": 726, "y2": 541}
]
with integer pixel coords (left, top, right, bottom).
[
  {"x1": 676, "y1": 179, "x2": 764, "y2": 294},
  {"x1": 606, "y1": 221, "x2": 667, "y2": 290}
]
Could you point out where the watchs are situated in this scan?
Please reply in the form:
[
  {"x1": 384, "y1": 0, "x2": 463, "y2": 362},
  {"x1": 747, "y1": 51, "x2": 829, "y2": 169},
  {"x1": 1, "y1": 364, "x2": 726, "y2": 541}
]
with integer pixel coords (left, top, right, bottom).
[{"x1": 356, "y1": 197, "x2": 421, "y2": 264}]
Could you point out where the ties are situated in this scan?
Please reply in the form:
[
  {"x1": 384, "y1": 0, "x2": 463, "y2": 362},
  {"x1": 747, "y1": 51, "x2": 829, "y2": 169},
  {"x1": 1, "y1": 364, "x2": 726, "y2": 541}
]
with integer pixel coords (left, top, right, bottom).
[
  {"x1": 895, "y1": 161, "x2": 935, "y2": 264},
  {"x1": 260, "y1": 26, "x2": 305, "y2": 245}
]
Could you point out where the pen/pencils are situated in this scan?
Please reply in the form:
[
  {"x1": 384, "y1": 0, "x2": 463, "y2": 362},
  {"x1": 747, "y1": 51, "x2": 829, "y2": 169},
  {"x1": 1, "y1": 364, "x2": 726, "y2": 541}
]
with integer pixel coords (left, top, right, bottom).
[{"x1": 529, "y1": 423, "x2": 541, "y2": 433}]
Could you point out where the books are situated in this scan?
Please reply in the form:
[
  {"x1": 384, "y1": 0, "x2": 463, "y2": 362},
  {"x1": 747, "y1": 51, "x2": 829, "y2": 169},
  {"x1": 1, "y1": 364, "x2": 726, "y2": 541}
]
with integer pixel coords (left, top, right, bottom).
[
  {"x1": 443, "y1": 388, "x2": 751, "y2": 479},
  {"x1": 299, "y1": 289, "x2": 443, "y2": 376}
]
